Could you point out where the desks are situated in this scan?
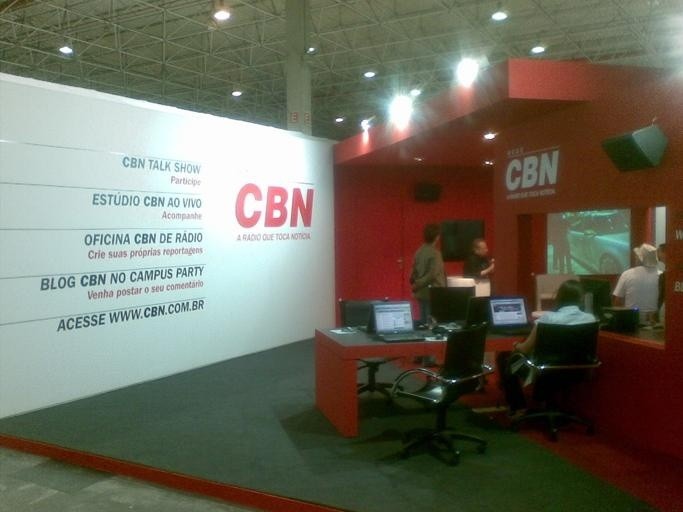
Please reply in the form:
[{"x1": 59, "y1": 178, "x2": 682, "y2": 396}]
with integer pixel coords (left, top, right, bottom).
[
  {"x1": 389, "y1": 326, "x2": 603, "y2": 466},
  {"x1": 313, "y1": 320, "x2": 530, "y2": 438}
]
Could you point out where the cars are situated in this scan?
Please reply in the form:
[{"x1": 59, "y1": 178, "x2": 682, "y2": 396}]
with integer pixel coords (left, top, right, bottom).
[{"x1": 559, "y1": 208, "x2": 633, "y2": 278}]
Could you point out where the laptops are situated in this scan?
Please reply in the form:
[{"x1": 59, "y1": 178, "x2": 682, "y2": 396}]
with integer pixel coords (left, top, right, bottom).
[
  {"x1": 489, "y1": 296, "x2": 530, "y2": 335},
  {"x1": 359, "y1": 301, "x2": 374, "y2": 332},
  {"x1": 372, "y1": 300, "x2": 425, "y2": 342}
]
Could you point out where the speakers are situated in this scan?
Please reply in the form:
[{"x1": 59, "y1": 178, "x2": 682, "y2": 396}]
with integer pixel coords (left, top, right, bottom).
[
  {"x1": 598, "y1": 124, "x2": 668, "y2": 171},
  {"x1": 415, "y1": 184, "x2": 441, "y2": 201}
]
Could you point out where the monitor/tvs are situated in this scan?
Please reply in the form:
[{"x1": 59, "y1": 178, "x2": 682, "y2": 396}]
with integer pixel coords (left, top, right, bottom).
[
  {"x1": 430, "y1": 286, "x2": 475, "y2": 321},
  {"x1": 602, "y1": 307, "x2": 640, "y2": 338},
  {"x1": 464, "y1": 296, "x2": 490, "y2": 327}
]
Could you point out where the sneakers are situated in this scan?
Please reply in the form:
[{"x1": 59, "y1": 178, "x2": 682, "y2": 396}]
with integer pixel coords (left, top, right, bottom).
[{"x1": 507, "y1": 408, "x2": 528, "y2": 423}]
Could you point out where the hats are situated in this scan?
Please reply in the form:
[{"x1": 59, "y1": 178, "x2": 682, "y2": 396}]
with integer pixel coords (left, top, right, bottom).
[{"x1": 633, "y1": 243, "x2": 659, "y2": 267}]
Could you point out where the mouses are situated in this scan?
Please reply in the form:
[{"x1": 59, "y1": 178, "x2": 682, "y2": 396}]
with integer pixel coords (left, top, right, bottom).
[{"x1": 436, "y1": 333, "x2": 444, "y2": 340}]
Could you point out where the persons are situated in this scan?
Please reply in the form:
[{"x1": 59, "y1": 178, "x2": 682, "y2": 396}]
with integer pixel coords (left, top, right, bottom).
[
  {"x1": 613, "y1": 241, "x2": 664, "y2": 328},
  {"x1": 555, "y1": 222, "x2": 574, "y2": 275},
  {"x1": 551, "y1": 218, "x2": 571, "y2": 270},
  {"x1": 409, "y1": 222, "x2": 446, "y2": 368},
  {"x1": 646, "y1": 242, "x2": 666, "y2": 324},
  {"x1": 496, "y1": 279, "x2": 597, "y2": 422},
  {"x1": 463, "y1": 237, "x2": 497, "y2": 286}
]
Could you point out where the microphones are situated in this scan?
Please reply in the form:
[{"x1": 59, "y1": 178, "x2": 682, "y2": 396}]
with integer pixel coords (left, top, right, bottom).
[{"x1": 490, "y1": 258, "x2": 495, "y2": 274}]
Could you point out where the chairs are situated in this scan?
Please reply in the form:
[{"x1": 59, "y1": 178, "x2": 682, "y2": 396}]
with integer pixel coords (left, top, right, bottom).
[{"x1": 339, "y1": 284, "x2": 476, "y2": 397}]
